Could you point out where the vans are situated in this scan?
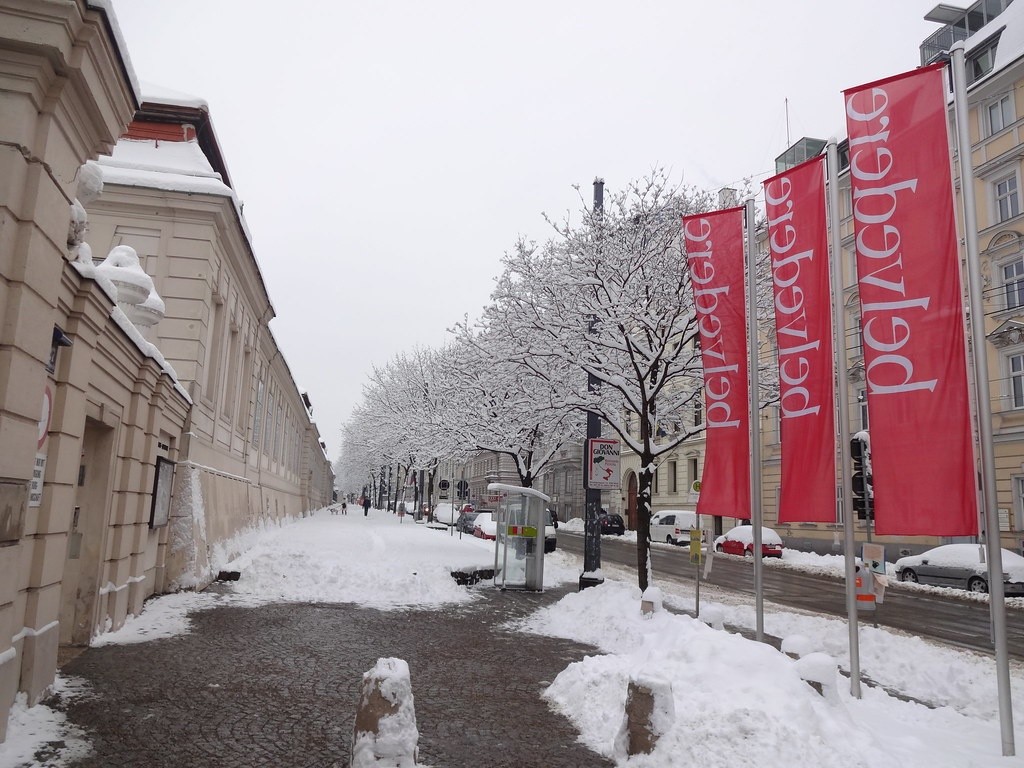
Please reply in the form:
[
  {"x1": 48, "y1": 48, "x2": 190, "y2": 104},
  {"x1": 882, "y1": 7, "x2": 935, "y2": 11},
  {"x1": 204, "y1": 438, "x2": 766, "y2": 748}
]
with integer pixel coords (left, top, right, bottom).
[
  {"x1": 498, "y1": 505, "x2": 558, "y2": 554},
  {"x1": 649, "y1": 510, "x2": 706, "y2": 546}
]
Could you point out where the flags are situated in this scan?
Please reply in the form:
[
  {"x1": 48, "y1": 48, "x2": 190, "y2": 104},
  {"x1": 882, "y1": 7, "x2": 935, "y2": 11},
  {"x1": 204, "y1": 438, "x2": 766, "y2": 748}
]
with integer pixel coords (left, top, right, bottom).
[
  {"x1": 843, "y1": 61, "x2": 980, "y2": 537},
  {"x1": 682, "y1": 207, "x2": 752, "y2": 518},
  {"x1": 763, "y1": 156, "x2": 836, "y2": 522}
]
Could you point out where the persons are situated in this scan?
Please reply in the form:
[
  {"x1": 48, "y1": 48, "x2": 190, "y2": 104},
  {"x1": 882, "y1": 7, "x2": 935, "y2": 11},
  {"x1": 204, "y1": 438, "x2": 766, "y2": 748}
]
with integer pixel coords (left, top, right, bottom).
[
  {"x1": 342, "y1": 501, "x2": 347, "y2": 515},
  {"x1": 362, "y1": 495, "x2": 371, "y2": 516}
]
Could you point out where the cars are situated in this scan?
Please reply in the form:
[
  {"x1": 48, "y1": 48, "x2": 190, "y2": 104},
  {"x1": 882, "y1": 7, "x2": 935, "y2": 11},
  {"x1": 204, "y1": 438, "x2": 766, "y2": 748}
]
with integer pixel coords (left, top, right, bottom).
[
  {"x1": 358, "y1": 497, "x2": 498, "y2": 542},
  {"x1": 714, "y1": 526, "x2": 782, "y2": 559},
  {"x1": 894, "y1": 544, "x2": 1024, "y2": 597},
  {"x1": 597, "y1": 514, "x2": 625, "y2": 536}
]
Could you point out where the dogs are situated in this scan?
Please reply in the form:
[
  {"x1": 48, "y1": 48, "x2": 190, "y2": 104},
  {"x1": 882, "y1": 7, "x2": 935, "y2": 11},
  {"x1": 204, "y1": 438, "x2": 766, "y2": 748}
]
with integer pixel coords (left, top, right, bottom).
[{"x1": 326, "y1": 507, "x2": 340, "y2": 515}]
